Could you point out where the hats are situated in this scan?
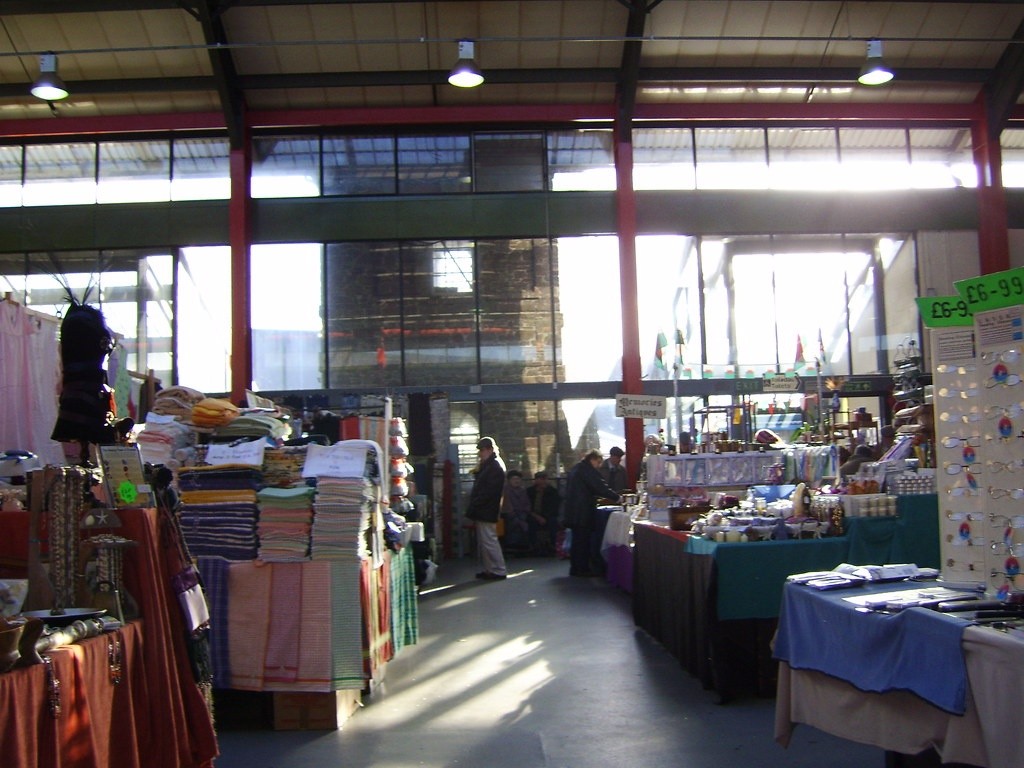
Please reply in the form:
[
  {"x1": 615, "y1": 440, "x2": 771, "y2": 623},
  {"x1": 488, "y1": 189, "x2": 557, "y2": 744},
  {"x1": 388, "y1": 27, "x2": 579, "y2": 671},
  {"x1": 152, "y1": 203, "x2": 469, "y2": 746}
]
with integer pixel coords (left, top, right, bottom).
[
  {"x1": 881, "y1": 425, "x2": 897, "y2": 437},
  {"x1": 476, "y1": 437, "x2": 496, "y2": 449},
  {"x1": 853, "y1": 444, "x2": 874, "y2": 461},
  {"x1": 610, "y1": 447, "x2": 624, "y2": 456}
]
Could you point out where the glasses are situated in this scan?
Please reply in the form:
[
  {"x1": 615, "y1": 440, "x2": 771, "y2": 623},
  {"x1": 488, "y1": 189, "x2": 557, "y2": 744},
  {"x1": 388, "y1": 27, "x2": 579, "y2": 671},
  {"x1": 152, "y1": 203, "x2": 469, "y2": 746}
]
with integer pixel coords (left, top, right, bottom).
[{"x1": 936, "y1": 348, "x2": 1024, "y2": 594}]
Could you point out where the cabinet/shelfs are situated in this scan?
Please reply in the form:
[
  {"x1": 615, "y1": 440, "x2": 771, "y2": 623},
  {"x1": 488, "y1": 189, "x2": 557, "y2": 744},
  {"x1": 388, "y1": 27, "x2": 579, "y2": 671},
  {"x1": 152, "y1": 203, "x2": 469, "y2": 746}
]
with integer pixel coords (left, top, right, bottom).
[{"x1": 829, "y1": 412, "x2": 879, "y2": 447}]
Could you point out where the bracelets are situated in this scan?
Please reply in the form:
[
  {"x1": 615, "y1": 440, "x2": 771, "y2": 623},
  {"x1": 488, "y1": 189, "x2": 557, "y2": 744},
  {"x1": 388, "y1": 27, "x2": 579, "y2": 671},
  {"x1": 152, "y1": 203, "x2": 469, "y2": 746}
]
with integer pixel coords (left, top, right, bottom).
[
  {"x1": 44, "y1": 656, "x2": 62, "y2": 720},
  {"x1": 108, "y1": 629, "x2": 122, "y2": 684}
]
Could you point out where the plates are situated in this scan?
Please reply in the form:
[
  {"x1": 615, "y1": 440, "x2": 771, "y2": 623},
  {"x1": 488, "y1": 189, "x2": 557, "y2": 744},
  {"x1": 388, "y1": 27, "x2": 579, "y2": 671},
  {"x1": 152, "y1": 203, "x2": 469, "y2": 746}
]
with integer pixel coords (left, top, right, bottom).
[{"x1": 18, "y1": 607, "x2": 106, "y2": 628}]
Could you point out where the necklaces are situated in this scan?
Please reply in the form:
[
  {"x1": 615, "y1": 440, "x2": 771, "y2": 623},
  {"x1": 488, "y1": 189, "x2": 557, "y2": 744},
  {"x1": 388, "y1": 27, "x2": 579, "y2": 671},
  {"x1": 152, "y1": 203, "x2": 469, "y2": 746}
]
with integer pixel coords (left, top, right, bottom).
[{"x1": 48, "y1": 465, "x2": 124, "y2": 611}]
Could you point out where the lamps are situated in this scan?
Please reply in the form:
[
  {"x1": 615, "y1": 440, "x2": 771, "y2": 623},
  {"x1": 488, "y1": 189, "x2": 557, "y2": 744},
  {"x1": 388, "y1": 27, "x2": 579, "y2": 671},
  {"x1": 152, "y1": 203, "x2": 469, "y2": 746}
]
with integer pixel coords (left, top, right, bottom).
[
  {"x1": 448, "y1": 41, "x2": 485, "y2": 88},
  {"x1": 858, "y1": 41, "x2": 895, "y2": 85},
  {"x1": 29, "y1": 54, "x2": 70, "y2": 100}
]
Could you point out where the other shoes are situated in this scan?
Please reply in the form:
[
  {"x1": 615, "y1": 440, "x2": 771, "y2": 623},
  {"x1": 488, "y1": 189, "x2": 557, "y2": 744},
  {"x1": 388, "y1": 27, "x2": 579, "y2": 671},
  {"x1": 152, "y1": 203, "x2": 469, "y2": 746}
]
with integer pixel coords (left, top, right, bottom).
[
  {"x1": 577, "y1": 568, "x2": 599, "y2": 576},
  {"x1": 475, "y1": 572, "x2": 506, "y2": 580}
]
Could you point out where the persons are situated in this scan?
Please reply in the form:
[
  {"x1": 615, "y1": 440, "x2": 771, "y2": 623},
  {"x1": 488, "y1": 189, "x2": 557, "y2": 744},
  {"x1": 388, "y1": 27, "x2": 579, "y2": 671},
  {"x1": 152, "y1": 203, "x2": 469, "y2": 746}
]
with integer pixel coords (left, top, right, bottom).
[
  {"x1": 565, "y1": 447, "x2": 628, "y2": 577},
  {"x1": 839, "y1": 425, "x2": 897, "y2": 475},
  {"x1": 680, "y1": 432, "x2": 692, "y2": 452},
  {"x1": 465, "y1": 437, "x2": 507, "y2": 580},
  {"x1": 502, "y1": 470, "x2": 561, "y2": 557}
]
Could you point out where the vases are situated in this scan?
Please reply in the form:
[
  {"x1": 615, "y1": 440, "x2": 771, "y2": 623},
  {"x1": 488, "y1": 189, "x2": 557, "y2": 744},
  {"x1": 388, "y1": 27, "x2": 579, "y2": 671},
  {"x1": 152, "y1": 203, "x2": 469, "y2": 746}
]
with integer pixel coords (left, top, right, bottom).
[{"x1": 832, "y1": 389, "x2": 841, "y2": 411}]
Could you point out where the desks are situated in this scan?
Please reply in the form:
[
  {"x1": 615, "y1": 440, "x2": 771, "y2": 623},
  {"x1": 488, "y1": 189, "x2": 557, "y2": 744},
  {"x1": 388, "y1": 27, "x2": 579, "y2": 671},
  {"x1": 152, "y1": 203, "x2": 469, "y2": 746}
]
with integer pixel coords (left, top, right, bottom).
[
  {"x1": 594, "y1": 496, "x2": 1024, "y2": 768},
  {"x1": 0, "y1": 505, "x2": 420, "y2": 768}
]
